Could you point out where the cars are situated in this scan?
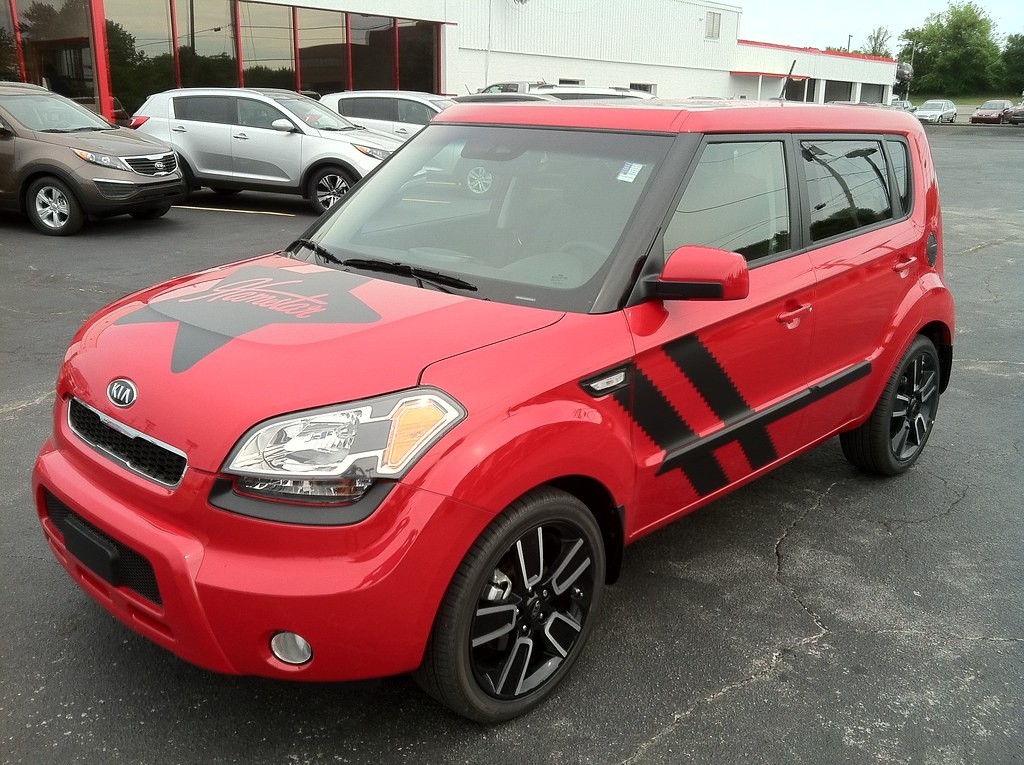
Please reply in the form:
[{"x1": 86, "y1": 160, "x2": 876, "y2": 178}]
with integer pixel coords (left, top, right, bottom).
[
  {"x1": 911, "y1": 100, "x2": 959, "y2": 123},
  {"x1": 890, "y1": 101, "x2": 916, "y2": 112},
  {"x1": 1003, "y1": 101, "x2": 1024, "y2": 125},
  {"x1": 971, "y1": 100, "x2": 1014, "y2": 124}
]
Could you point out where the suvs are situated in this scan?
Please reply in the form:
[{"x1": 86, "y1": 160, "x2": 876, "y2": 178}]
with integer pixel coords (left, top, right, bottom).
[
  {"x1": 451, "y1": 80, "x2": 660, "y2": 101},
  {"x1": 126, "y1": 87, "x2": 408, "y2": 215},
  {"x1": 314, "y1": 91, "x2": 552, "y2": 201},
  {"x1": 0, "y1": 81, "x2": 185, "y2": 237},
  {"x1": 28, "y1": 100, "x2": 954, "y2": 722}
]
